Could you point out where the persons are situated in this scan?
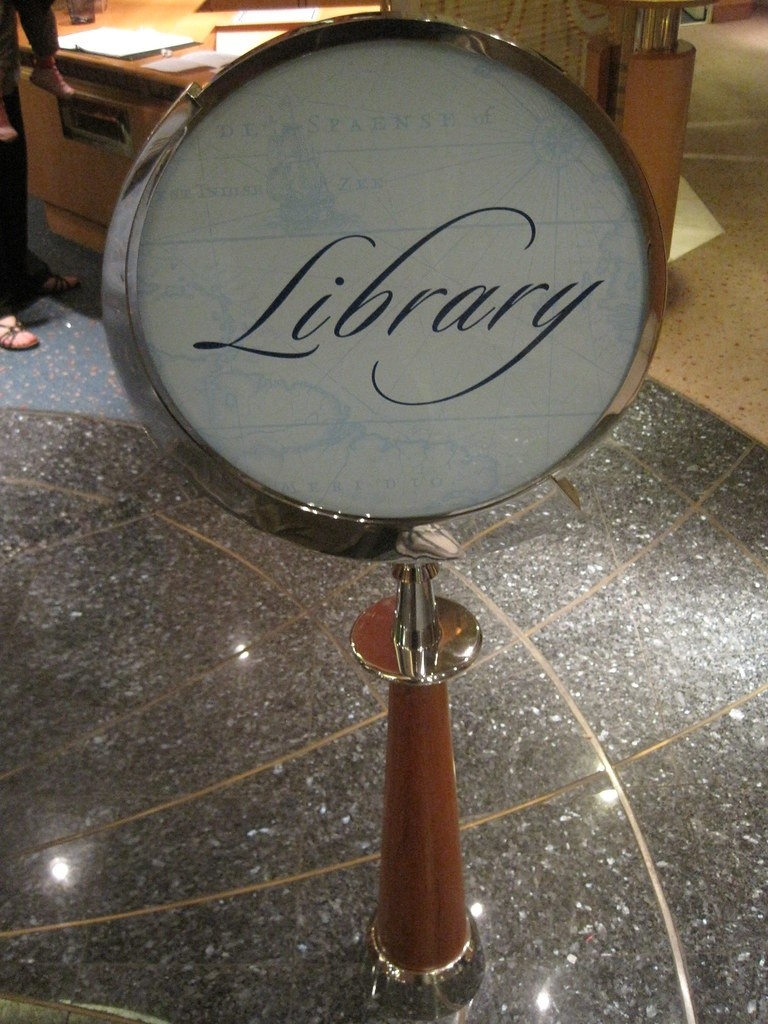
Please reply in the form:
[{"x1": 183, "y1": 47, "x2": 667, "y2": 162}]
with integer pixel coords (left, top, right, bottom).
[
  {"x1": 0, "y1": 0, "x2": 76, "y2": 141},
  {"x1": 0, "y1": 0, "x2": 79, "y2": 350}
]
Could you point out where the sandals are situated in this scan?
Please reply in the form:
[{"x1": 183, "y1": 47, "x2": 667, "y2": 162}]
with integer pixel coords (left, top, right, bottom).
[
  {"x1": 0, "y1": 315, "x2": 40, "y2": 350},
  {"x1": 42, "y1": 275, "x2": 80, "y2": 290}
]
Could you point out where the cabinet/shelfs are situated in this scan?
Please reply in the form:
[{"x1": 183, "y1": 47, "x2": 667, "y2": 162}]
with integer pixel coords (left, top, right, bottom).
[{"x1": 0, "y1": 0, "x2": 391, "y2": 256}]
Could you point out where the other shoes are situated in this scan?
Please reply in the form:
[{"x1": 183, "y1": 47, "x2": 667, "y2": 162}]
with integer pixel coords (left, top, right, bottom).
[
  {"x1": 30, "y1": 61, "x2": 76, "y2": 100},
  {"x1": 0, "y1": 106, "x2": 18, "y2": 141}
]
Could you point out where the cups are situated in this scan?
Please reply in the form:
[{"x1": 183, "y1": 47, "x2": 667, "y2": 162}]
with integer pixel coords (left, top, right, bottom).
[{"x1": 65, "y1": 0, "x2": 95, "y2": 24}]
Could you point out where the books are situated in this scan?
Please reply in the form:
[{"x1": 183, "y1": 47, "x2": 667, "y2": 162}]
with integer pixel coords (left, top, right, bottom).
[{"x1": 56, "y1": 26, "x2": 203, "y2": 62}]
